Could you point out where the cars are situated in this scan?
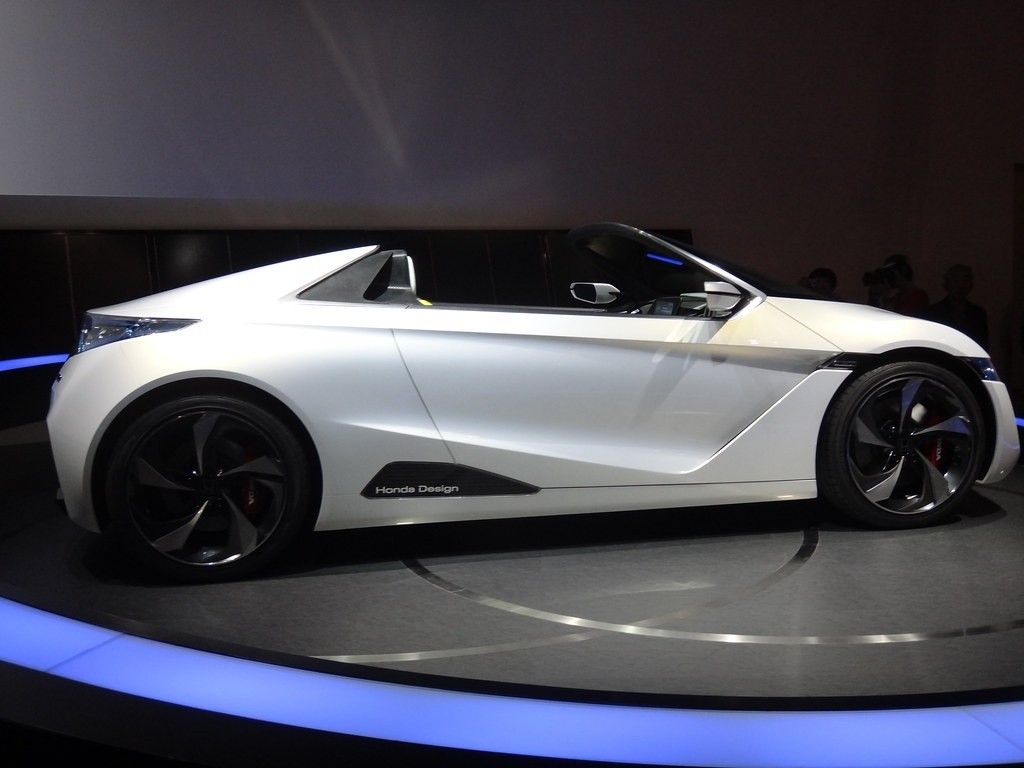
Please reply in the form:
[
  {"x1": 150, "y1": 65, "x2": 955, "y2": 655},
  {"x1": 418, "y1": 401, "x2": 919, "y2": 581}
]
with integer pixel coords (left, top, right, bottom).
[{"x1": 47, "y1": 220, "x2": 1021, "y2": 582}]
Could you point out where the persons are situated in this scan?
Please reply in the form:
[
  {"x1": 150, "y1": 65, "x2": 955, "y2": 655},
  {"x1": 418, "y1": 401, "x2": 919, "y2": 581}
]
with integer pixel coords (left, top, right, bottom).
[
  {"x1": 807, "y1": 267, "x2": 836, "y2": 292},
  {"x1": 925, "y1": 267, "x2": 991, "y2": 349},
  {"x1": 862, "y1": 254, "x2": 930, "y2": 314}
]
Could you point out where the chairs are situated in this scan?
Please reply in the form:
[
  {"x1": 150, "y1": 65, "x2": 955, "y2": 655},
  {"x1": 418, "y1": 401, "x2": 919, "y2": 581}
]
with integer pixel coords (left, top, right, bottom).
[{"x1": 382, "y1": 251, "x2": 436, "y2": 302}]
